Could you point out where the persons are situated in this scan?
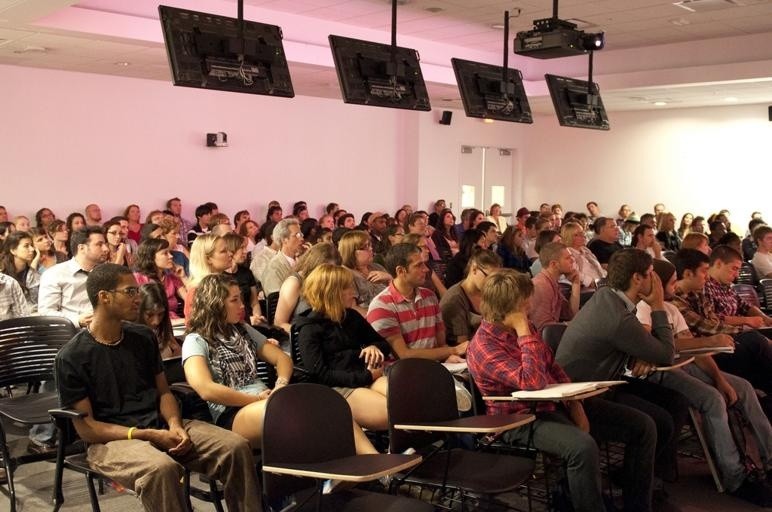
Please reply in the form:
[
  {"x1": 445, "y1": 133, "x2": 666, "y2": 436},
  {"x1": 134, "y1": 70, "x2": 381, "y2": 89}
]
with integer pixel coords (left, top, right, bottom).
[
  {"x1": 368, "y1": 243, "x2": 475, "y2": 411},
  {"x1": 436, "y1": 246, "x2": 506, "y2": 347},
  {"x1": 468, "y1": 268, "x2": 657, "y2": 512},
  {"x1": 585, "y1": 198, "x2": 772, "y2": 286},
  {"x1": 182, "y1": 271, "x2": 382, "y2": 490},
  {"x1": 338, "y1": 197, "x2": 609, "y2": 286},
  {"x1": 556, "y1": 248, "x2": 681, "y2": 512},
  {"x1": 524, "y1": 242, "x2": 581, "y2": 336},
  {"x1": 636, "y1": 261, "x2": 772, "y2": 506},
  {"x1": 54, "y1": 264, "x2": 260, "y2": 512},
  {"x1": 700, "y1": 246, "x2": 772, "y2": 360},
  {"x1": 0, "y1": 195, "x2": 367, "y2": 327},
  {"x1": 667, "y1": 248, "x2": 772, "y2": 414},
  {"x1": 296, "y1": 265, "x2": 389, "y2": 433}
]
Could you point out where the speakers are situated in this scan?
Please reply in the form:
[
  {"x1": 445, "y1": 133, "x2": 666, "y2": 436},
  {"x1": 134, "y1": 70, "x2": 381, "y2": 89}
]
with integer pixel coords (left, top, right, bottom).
[{"x1": 442, "y1": 111, "x2": 452, "y2": 126}]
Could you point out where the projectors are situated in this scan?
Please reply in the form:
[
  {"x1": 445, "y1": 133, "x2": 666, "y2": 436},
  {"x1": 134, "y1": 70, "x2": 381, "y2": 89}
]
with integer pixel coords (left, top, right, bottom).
[{"x1": 514, "y1": 18, "x2": 605, "y2": 59}]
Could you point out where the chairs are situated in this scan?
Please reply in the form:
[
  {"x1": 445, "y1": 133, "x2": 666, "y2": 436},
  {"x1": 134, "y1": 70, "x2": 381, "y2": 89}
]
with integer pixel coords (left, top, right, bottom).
[
  {"x1": 661, "y1": 250, "x2": 675, "y2": 261},
  {"x1": 0, "y1": 316, "x2": 103, "y2": 512},
  {"x1": 760, "y1": 279, "x2": 772, "y2": 312},
  {"x1": 736, "y1": 262, "x2": 755, "y2": 282},
  {"x1": 164, "y1": 352, "x2": 314, "y2": 512},
  {"x1": 261, "y1": 383, "x2": 435, "y2": 512},
  {"x1": 48, "y1": 360, "x2": 194, "y2": 512},
  {"x1": 734, "y1": 284, "x2": 762, "y2": 315},
  {"x1": 540, "y1": 322, "x2": 569, "y2": 356},
  {"x1": 387, "y1": 357, "x2": 537, "y2": 512},
  {"x1": 465, "y1": 342, "x2": 614, "y2": 512},
  {"x1": 170, "y1": 380, "x2": 217, "y2": 423}
]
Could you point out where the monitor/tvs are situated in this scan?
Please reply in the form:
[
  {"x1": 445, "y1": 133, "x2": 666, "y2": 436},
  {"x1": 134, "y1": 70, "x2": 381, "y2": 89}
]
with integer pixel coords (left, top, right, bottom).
[
  {"x1": 544, "y1": 73, "x2": 610, "y2": 131},
  {"x1": 450, "y1": 58, "x2": 534, "y2": 124},
  {"x1": 157, "y1": 4, "x2": 295, "y2": 98},
  {"x1": 328, "y1": 34, "x2": 432, "y2": 112}
]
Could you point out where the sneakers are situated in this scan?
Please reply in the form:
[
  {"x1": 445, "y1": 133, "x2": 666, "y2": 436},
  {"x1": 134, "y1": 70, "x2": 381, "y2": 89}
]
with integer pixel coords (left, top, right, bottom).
[
  {"x1": 725, "y1": 478, "x2": 771, "y2": 507},
  {"x1": 654, "y1": 487, "x2": 669, "y2": 502}
]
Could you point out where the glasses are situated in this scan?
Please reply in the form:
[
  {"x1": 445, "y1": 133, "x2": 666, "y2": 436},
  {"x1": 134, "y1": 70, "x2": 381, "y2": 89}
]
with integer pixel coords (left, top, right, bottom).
[
  {"x1": 395, "y1": 232, "x2": 405, "y2": 236},
  {"x1": 291, "y1": 232, "x2": 304, "y2": 239},
  {"x1": 355, "y1": 240, "x2": 373, "y2": 250},
  {"x1": 107, "y1": 286, "x2": 140, "y2": 298}
]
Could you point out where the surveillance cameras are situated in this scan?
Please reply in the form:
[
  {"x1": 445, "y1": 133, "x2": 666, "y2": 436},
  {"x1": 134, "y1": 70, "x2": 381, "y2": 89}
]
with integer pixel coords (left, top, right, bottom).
[{"x1": 206, "y1": 131, "x2": 229, "y2": 147}]
